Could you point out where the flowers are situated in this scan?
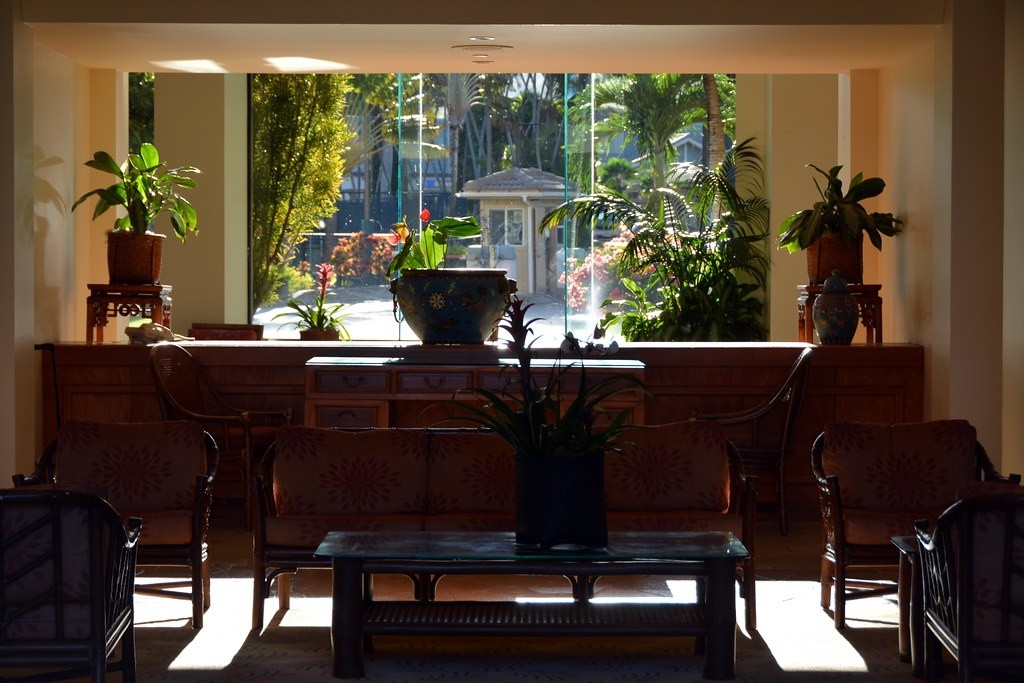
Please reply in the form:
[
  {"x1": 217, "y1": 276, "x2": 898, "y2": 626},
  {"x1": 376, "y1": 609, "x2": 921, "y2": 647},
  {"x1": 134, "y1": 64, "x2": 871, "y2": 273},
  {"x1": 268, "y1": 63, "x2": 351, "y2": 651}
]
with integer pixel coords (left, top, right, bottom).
[
  {"x1": 385, "y1": 207, "x2": 483, "y2": 280},
  {"x1": 273, "y1": 264, "x2": 350, "y2": 340}
]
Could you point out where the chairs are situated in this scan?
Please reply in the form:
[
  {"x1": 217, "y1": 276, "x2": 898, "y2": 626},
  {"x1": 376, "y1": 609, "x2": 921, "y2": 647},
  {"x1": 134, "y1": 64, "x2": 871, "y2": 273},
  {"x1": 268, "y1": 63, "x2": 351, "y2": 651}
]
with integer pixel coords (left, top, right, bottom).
[{"x1": 0, "y1": 346, "x2": 1024, "y2": 683}]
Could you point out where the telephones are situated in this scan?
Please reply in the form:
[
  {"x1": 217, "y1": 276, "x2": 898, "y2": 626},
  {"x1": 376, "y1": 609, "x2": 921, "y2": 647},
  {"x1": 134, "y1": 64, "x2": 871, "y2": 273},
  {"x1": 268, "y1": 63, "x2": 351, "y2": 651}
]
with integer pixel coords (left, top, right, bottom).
[{"x1": 125, "y1": 323, "x2": 175, "y2": 344}]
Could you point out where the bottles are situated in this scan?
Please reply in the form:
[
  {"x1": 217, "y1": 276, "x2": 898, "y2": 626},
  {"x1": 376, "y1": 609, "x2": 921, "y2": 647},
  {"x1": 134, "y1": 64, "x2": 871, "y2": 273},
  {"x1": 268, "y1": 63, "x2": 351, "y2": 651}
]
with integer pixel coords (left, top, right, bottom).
[
  {"x1": 155, "y1": 285, "x2": 173, "y2": 330},
  {"x1": 798, "y1": 290, "x2": 811, "y2": 343}
]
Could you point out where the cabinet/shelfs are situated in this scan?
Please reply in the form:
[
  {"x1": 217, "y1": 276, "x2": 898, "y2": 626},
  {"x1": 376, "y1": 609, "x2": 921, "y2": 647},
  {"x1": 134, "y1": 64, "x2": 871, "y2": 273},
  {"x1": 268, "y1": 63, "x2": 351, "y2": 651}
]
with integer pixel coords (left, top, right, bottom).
[{"x1": 304, "y1": 355, "x2": 649, "y2": 430}]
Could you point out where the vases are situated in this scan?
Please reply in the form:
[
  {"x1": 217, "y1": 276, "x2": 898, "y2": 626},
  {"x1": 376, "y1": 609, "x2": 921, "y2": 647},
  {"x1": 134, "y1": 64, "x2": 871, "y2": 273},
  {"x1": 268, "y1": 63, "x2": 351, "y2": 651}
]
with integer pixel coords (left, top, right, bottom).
[
  {"x1": 301, "y1": 330, "x2": 339, "y2": 340},
  {"x1": 390, "y1": 268, "x2": 518, "y2": 345}
]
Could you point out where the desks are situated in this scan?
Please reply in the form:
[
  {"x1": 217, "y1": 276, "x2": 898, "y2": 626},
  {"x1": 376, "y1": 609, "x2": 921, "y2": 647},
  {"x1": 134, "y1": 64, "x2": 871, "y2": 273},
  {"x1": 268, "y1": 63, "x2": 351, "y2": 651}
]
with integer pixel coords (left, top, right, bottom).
[
  {"x1": 794, "y1": 284, "x2": 883, "y2": 342},
  {"x1": 890, "y1": 533, "x2": 934, "y2": 667},
  {"x1": 312, "y1": 529, "x2": 753, "y2": 681},
  {"x1": 84, "y1": 282, "x2": 174, "y2": 341}
]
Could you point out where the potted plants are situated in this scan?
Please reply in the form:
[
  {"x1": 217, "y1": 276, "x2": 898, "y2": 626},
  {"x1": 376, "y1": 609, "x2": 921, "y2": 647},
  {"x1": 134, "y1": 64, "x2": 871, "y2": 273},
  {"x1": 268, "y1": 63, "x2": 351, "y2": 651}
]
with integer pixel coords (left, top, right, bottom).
[
  {"x1": 776, "y1": 160, "x2": 906, "y2": 284},
  {"x1": 420, "y1": 293, "x2": 659, "y2": 547},
  {"x1": 70, "y1": 142, "x2": 202, "y2": 281}
]
